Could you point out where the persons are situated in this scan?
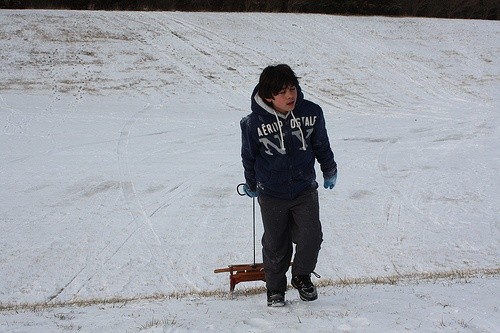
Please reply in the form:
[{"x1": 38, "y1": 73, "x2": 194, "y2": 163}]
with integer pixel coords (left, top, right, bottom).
[{"x1": 239, "y1": 64, "x2": 337, "y2": 308}]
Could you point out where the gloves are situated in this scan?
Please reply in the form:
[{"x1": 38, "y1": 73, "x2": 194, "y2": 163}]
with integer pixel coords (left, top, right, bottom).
[
  {"x1": 242, "y1": 183, "x2": 258, "y2": 198},
  {"x1": 324, "y1": 172, "x2": 337, "y2": 190}
]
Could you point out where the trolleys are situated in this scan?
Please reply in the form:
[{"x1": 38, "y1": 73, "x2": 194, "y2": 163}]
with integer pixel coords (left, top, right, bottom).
[{"x1": 213, "y1": 197, "x2": 266, "y2": 292}]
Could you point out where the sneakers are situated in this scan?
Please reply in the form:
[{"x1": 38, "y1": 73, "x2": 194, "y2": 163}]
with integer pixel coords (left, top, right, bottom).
[
  {"x1": 291, "y1": 274, "x2": 317, "y2": 302},
  {"x1": 266, "y1": 288, "x2": 286, "y2": 307}
]
